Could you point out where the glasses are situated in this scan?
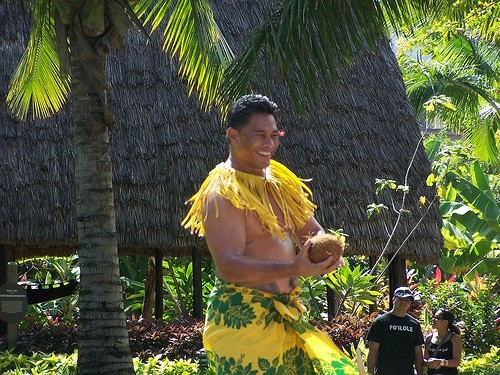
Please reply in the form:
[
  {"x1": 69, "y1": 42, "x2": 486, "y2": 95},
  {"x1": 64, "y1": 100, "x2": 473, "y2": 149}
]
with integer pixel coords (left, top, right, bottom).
[{"x1": 432, "y1": 315, "x2": 447, "y2": 322}]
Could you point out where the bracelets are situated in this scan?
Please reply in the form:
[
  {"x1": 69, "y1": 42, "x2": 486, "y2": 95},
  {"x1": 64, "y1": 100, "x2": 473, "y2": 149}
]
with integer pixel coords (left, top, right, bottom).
[{"x1": 439, "y1": 359, "x2": 449, "y2": 367}]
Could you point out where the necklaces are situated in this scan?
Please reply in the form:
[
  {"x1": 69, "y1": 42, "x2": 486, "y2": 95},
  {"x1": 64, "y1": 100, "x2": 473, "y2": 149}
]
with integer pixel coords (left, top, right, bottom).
[{"x1": 436, "y1": 331, "x2": 449, "y2": 344}]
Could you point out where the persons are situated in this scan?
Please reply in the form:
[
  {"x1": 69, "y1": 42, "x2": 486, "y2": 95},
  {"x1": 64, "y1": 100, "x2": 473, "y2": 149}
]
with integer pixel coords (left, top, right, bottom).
[
  {"x1": 366, "y1": 285, "x2": 426, "y2": 375},
  {"x1": 181, "y1": 92, "x2": 364, "y2": 375},
  {"x1": 421, "y1": 306, "x2": 464, "y2": 375}
]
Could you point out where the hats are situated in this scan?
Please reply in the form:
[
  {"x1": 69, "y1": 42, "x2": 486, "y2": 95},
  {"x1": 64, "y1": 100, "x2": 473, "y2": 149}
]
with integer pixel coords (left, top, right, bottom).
[{"x1": 393, "y1": 286, "x2": 415, "y2": 301}]
